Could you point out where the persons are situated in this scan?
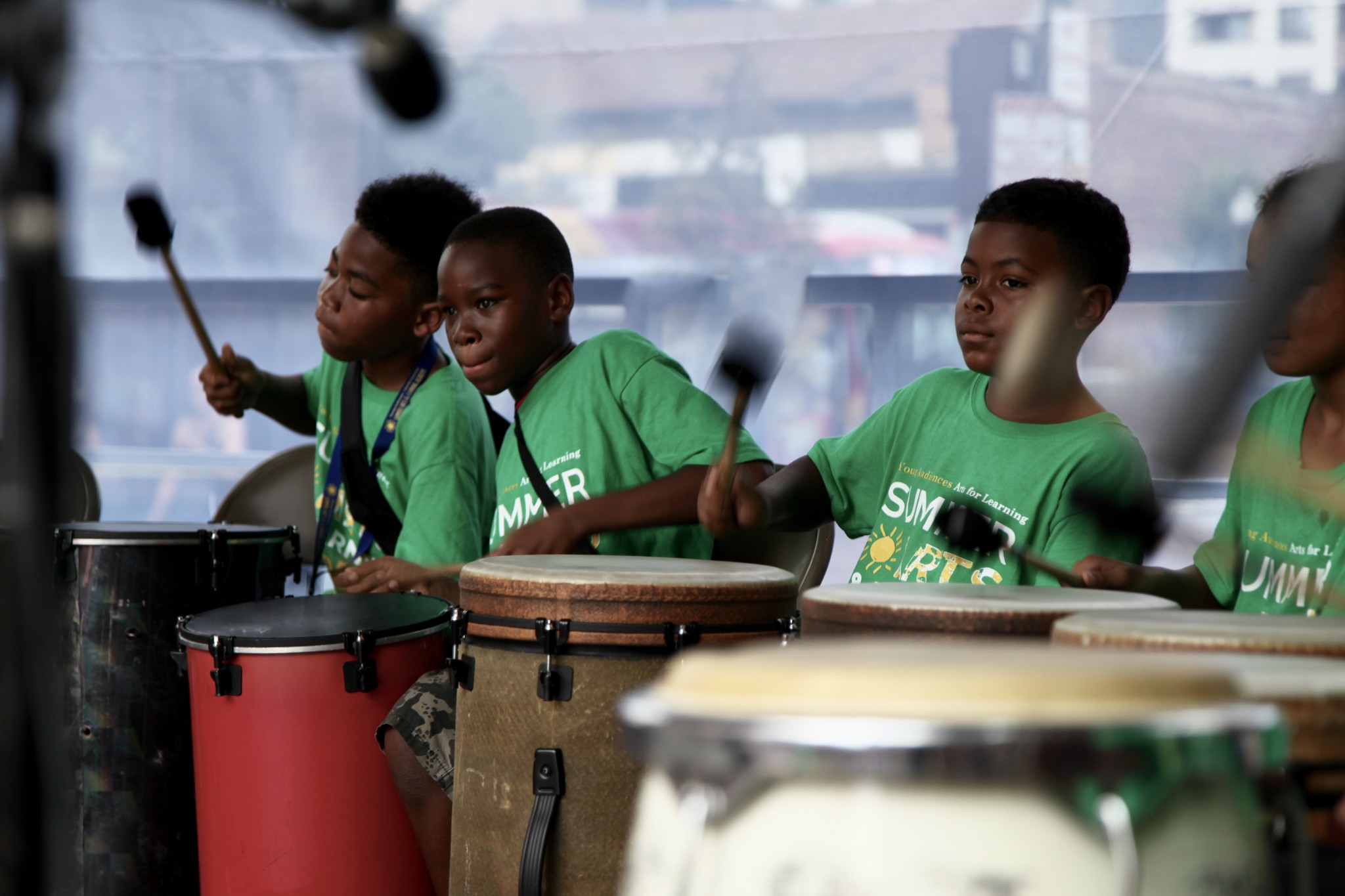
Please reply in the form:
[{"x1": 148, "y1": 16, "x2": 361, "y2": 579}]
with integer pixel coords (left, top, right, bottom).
[
  {"x1": 1058, "y1": 160, "x2": 1345, "y2": 620},
  {"x1": 697, "y1": 176, "x2": 1167, "y2": 586},
  {"x1": 333, "y1": 207, "x2": 775, "y2": 596},
  {"x1": 198, "y1": 171, "x2": 497, "y2": 611}
]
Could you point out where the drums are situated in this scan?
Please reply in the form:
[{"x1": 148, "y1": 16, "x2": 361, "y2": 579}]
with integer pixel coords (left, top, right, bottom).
[
  {"x1": 452, "y1": 552, "x2": 802, "y2": 895},
  {"x1": 801, "y1": 581, "x2": 1176, "y2": 639},
  {"x1": 173, "y1": 589, "x2": 451, "y2": 896},
  {"x1": 1051, "y1": 609, "x2": 1345, "y2": 896},
  {"x1": 50, "y1": 520, "x2": 293, "y2": 895},
  {"x1": 620, "y1": 632, "x2": 1285, "y2": 895}
]
